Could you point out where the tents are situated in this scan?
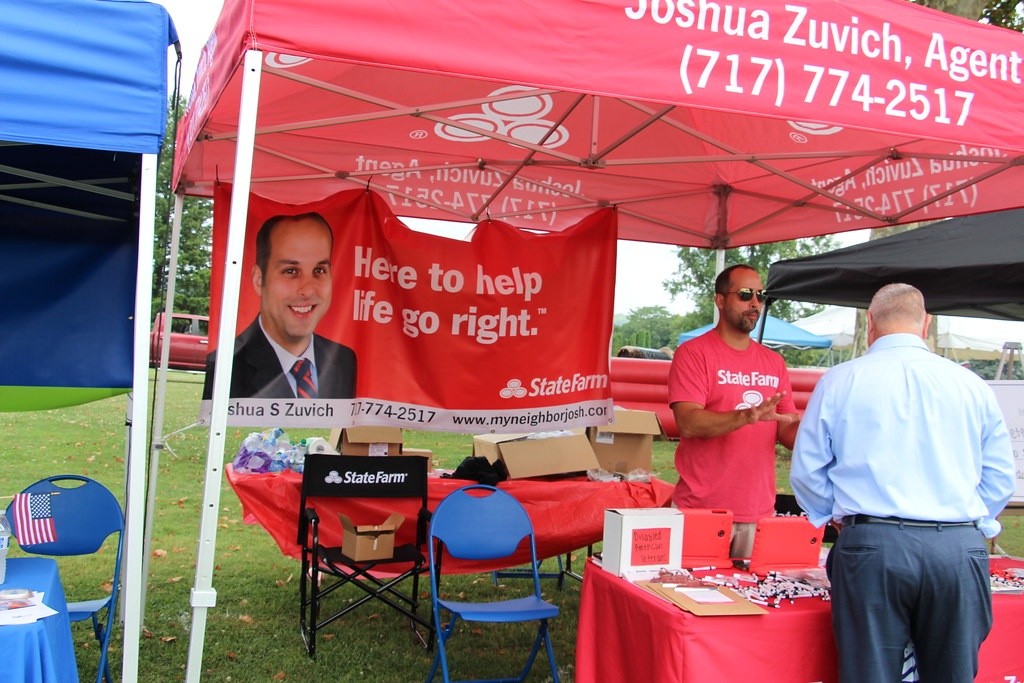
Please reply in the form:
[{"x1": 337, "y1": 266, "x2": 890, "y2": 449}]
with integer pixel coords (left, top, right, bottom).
[
  {"x1": 0, "y1": 0, "x2": 182, "y2": 683},
  {"x1": 141, "y1": 0, "x2": 1024, "y2": 683},
  {"x1": 679, "y1": 315, "x2": 832, "y2": 367},
  {"x1": 759, "y1": 207, "x2": 1024, "y2": 347}
]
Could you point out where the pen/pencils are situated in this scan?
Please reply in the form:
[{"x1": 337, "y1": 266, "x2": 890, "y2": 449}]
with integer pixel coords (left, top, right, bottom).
[
  {"x1": 990, "y1": 569, "x2": 1024, "y2": 589},
  {"x1": 686, "y1": 559, "x2": 831, "y2": 609}
]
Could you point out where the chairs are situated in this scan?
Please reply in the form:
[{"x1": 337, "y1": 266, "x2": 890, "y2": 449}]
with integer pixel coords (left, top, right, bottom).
[
  {"x1": 4, "y1": 475, "x2": 126, "y2": 683},
  {"x1": 299, "y1": 453, "x2": 443, "y2": 658},
  {"x1": 423, "y1": 484, "x2": 562, "y2": 683},
  {"x1": 492, "y1": 554, "x2": 564, "y2": 588}
]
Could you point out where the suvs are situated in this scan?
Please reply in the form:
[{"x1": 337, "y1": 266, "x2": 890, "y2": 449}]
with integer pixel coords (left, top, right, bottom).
[{"x1": 149, "y1": 311, "x2": 208, "y2": 370}]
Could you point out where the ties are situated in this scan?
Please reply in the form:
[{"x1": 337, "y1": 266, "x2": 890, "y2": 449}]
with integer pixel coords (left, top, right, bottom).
[{"x1": 290, "y1": 359, "x2": 318, "y2": 398}]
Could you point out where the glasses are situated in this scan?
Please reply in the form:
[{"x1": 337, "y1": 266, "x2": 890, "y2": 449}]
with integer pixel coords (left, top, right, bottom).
[{"x1": 726, "y1": 287, "x2": 768, "y2": 301}]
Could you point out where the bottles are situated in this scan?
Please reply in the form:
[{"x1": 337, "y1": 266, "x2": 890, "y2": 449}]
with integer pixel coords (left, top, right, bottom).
[
  {"x1": 0, "y1": 510, "x2": 11, "y2": 585},
  {"x1": 255, "y1": 431, "x2": 307, "y2": 473}
]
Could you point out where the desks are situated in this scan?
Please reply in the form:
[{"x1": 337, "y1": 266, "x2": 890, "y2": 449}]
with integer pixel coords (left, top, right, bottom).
[
  {"x1": 224, "y1": 463, "x2": 675, "y2": 620},
  {"x1": 574, "y1": 556, "x2": 1024, "y2": 683},
  {"x1": 0, "y1": 557, "x2": 80, "y2": 683}
]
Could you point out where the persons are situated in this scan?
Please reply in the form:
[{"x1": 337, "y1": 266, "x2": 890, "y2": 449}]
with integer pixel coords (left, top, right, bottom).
[
  {"x1": 202, "y1": 211, "x2": 357, "y2": 399},
  {"x1": 669, "y1": 264, "x2": 804, "y2": 516},
  {"x1": 791, "y1": 282, "x2": 1016, "y2": 683}
]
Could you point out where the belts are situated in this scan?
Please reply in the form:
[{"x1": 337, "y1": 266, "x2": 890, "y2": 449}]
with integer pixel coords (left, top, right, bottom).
[{"x1": 842, "y1": 513, "x2": 976, "y2": 526}]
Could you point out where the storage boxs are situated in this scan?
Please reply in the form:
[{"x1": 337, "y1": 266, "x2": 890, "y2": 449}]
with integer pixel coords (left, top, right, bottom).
[
  {"x1": 328, "y1": 425, "x2": 433, "y2": 473},
  {"x1": 602, "y1": 507, "x2": 684, "y2": 579},
  {"x1": 586, "y1": 409, "x2": 670, "y2": 480},
  {"x1": 473, "y1": 426, "x2": 601, "y2": 479},
  {"x1": 336, "y1": 510, "x2": 406, "y2": 562}
]
